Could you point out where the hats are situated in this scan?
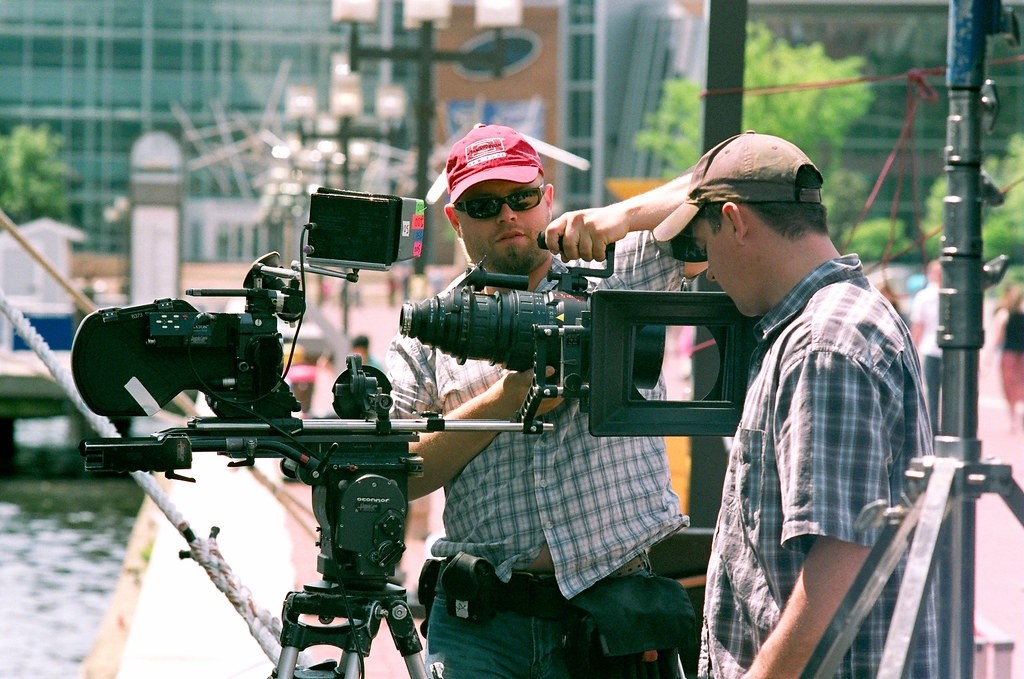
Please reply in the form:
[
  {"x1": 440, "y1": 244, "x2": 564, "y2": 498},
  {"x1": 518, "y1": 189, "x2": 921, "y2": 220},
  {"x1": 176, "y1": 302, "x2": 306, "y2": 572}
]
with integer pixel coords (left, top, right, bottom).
[
  {"x1": 651, "y1": 129, "x2": 823, "y2": 263},
  {"x1": 447, "y1": 123, "x2": 544, "y2": 203}
]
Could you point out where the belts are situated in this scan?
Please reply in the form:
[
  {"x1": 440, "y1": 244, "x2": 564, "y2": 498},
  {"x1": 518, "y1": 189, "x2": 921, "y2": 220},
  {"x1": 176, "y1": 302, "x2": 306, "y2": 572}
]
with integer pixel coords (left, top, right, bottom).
[{"x1": 495, "y1": 571, "x2": 578, "y2": 613}]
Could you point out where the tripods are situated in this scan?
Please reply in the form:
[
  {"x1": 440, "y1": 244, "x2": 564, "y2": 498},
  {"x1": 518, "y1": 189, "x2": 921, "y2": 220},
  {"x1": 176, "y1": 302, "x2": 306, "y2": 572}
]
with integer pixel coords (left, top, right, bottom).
[
  {"x1": 813, "y1": 0, "x2": 1024, "y2": 679},
  {"x1": 272, "y1": 449, "x2": 432, "y2": 679}
]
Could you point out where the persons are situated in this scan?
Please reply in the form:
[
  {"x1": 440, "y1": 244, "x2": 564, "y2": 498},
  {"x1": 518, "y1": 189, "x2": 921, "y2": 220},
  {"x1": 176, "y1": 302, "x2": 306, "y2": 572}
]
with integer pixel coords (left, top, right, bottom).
[
  {"x1": 909, "y1": 261, "x2": 943, "y2": 434},
  {"x1": 654, "y1": 131, "x2": 936, "y2": 679},
  {"x1": 77, "y1": 261, "x2": 914, "y2": 400},
  {"x1": 985, "y1": 283, "x2": 1024, "y2": 436},
  {"x1": 383, "y1": 125, "x2": 708, "y2": 679}
]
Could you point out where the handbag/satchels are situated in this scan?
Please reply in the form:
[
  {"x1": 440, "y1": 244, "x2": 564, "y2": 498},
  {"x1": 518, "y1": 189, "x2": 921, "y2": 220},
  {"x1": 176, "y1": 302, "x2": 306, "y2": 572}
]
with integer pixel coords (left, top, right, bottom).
[{"x1": 561, "y1": 578, "x2": 698, "y2": 679}]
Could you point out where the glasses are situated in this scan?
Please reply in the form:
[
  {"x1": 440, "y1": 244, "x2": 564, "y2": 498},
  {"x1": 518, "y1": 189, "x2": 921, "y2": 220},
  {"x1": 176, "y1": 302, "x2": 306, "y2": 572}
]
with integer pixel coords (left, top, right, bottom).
[{"x1": 456, "y1": 183, "x2": 545, "y2": 218}]
